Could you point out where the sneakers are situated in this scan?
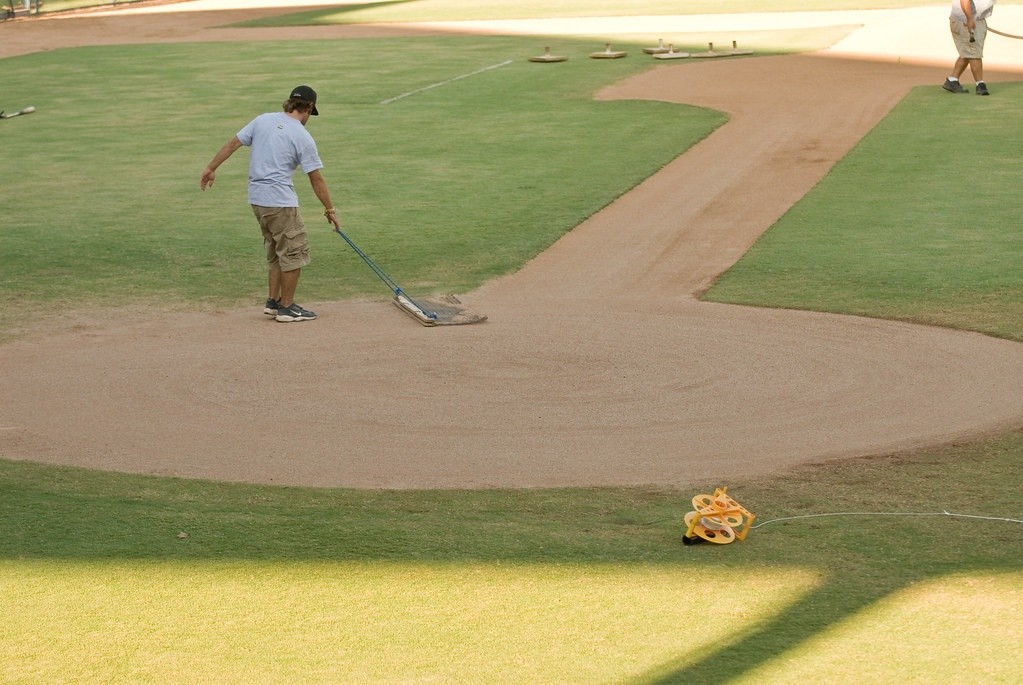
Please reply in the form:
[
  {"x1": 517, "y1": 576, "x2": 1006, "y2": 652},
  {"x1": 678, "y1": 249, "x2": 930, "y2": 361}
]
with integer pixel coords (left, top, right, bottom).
[
  {"x1": 276, "y1": 303, "x2": 317, "y2": 321},
  {"x1": 264, "y1": 297, "x2": 281, "y2": 316},
  {"x1": 976, "y1": 83, "x2": 989, "y2": 95},
  {"x1": 943, "y1": 77, "x2": 969, "y2": 93}
]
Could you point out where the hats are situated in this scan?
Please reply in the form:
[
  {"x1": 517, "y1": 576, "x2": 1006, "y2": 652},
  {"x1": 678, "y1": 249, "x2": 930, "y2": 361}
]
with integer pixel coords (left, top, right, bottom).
[{"x1": 290, "y1": 86, "x2": 318, "y2": 115}]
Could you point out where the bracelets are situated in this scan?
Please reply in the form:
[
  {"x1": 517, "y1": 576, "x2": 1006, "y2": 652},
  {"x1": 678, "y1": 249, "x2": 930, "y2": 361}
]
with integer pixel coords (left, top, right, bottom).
[{"x1": 325, "y1": 207, "x2": 335, "y2": 215}]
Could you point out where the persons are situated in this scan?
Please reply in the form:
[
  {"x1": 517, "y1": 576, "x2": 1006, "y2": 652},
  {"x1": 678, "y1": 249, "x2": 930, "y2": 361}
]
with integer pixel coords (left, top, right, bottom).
[
  {"x1": 942, "y1": 0, "x2": 995, "y2": 96},
  {"x1": 200, "y1": 85, "x2": 341, "y2": 322}
]
